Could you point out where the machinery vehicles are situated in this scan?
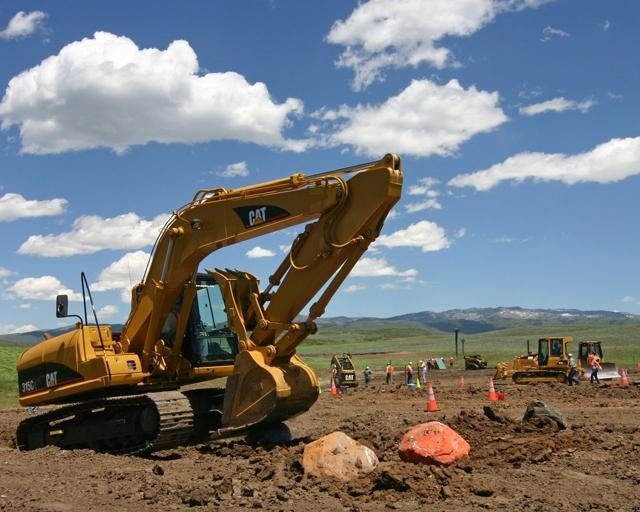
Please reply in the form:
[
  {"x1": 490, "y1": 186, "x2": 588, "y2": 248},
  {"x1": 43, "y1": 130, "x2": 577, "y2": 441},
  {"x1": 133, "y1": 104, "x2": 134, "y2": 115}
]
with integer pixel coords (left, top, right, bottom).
[
  {"x1": 324, "y1": 351, "x2": 363, "y2": 389},
  {"x1": 14, "y1": 154, "x2": 405, "y2": 459},
  {"x1": 497, "y1": 330, "x2": 620, "y2": 384}
]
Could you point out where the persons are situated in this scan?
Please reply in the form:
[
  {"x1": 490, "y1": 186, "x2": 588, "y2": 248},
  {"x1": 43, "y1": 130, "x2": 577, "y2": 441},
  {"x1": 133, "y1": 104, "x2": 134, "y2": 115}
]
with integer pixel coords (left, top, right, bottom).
[
  {"x1": 588, "y1": 355, "x2": 602, "y2": 383},
  {"x1": 567, "y1": 353, "x2": 581, "y2": 386},
  {"x1": 552, "y1": 341, "x2": 560, "y2": 355},
  {"x1": 362, "y1": 365, "x2": 373, "y2": 385},
  {"x1": 384, "y1": 362, "x2": 393, "y2": 384},
  {"x1": 449, "y1": 356, "x2": 454, "y2": 367},
  {"x1": 419, "y1": 360, "x2": 428, "y2": 385},
  {"x1": 330, "y1": 363, "x2": 340, "y2": 388},
  {"x1": 158, "y1": 302, "x2": 211, "y2": 364},
  {"x1": 406, "y1": 360, "x2": 413, "y2": 383}
]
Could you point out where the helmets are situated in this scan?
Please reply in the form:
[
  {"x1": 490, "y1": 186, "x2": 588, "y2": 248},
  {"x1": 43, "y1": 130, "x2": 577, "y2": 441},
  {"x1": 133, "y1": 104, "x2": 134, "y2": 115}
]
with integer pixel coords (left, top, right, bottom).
[
  {"x1": 419, "y1": 360, "x2": 422, "y2": 363},
  {"x1": 568, "y1": 354, "x2": 573, "y2": 357},
  {"x1": 408, "y1": 362, "x2": 412, "y2": 365},
  {"x1": 587, "y1": 355, "x2": 594, "y2": 360}
]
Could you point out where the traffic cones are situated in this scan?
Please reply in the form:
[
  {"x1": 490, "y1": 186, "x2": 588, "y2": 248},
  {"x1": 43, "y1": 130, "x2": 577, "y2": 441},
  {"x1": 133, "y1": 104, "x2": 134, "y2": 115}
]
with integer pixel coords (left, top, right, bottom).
[
  {"x1": 486, "y1": 375, "x2": 508, "y2": 402},
  {"x1": 609, "y1": 368, "x2": 628, "y2": 391},
  {"x1": 329, "y1": 378, "x2": 338, "y2": 396},
  {"x1": 457, "y1": 375, "x2": 467, "y2": 390},
  {"x1": 421, "y1": 382, "x2": 442, "y2": 413}
]
